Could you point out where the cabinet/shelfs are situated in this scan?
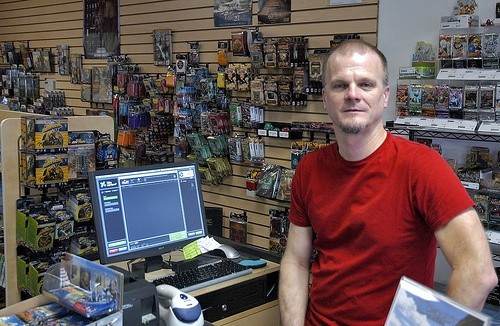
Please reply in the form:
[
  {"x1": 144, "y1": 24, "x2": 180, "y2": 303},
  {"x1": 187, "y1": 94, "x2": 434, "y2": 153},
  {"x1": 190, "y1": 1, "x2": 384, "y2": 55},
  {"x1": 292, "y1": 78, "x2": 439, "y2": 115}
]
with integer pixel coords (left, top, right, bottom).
[{"x1": 0, "y1": 107, "x2": 114, "y2": 307}]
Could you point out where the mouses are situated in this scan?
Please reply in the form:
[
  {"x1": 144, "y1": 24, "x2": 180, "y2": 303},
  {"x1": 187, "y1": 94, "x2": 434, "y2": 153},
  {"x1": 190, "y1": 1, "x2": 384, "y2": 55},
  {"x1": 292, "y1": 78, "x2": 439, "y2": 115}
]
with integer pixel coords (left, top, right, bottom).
[{"x1": 210, "y1": 245, "x2": 241, "y2": 260}]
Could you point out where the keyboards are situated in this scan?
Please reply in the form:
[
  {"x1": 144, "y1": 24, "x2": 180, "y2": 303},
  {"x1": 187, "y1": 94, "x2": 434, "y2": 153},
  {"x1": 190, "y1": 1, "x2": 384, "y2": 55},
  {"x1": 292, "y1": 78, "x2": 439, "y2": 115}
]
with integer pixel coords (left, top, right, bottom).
[{"x1": 150, "y1": 257, "x2": 252, "y2": 292}]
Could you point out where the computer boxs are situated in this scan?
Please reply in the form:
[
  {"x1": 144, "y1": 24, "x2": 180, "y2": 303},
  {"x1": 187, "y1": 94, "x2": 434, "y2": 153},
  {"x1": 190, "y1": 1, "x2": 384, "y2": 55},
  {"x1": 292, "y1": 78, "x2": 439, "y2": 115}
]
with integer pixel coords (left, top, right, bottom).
[{"x1": 105, "y1": 265, "x2": 161, "y2": 326}]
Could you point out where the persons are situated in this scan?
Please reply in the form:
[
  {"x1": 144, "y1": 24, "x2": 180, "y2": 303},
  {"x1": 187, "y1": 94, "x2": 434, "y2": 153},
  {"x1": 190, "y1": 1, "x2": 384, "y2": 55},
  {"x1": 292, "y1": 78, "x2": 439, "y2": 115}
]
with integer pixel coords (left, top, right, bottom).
[{"x1": 278, "y1": 39, "x2": 498, "y2": 326}]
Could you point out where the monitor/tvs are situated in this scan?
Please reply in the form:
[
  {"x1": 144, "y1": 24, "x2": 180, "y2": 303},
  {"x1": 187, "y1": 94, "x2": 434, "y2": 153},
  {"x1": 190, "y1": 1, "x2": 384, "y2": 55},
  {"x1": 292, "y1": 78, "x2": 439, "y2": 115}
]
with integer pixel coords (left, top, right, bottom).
[{"x1": 87, "y1": 160, "x2": 209, "y2": 264}]
